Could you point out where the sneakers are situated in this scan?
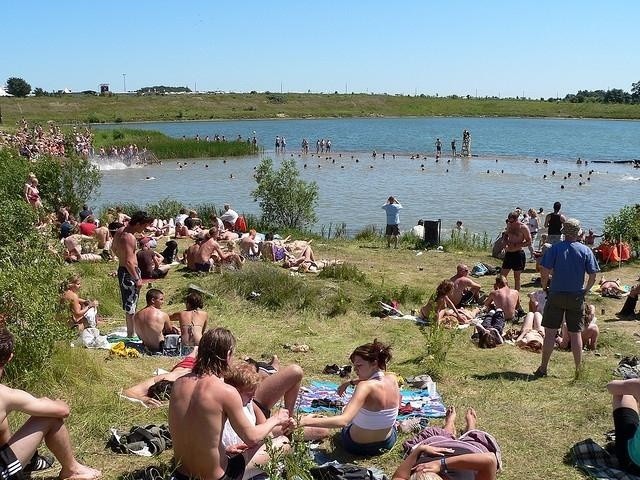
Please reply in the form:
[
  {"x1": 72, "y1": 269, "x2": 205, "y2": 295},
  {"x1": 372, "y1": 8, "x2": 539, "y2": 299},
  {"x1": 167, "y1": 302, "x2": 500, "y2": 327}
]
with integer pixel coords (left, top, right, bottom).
[
  {"x1": 25, "y1": 451, "x2": 54, "y2": 471},
  {"x1": 619, "y1": 356, "x2": 630, "y2": 365},
  {"x1": 340, "y1": 364, "x2": 352, "y2": 377},
  {"x1": 630, "y1": 356, "x2": 639, "y2": 365},
  {"x1": 322, "y1": 364, "x2": 339, "y2": 374}
]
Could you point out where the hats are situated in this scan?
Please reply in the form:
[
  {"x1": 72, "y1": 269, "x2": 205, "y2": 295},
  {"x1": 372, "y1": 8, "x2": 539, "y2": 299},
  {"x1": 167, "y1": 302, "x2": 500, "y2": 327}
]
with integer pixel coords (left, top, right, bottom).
[{"x1": 560, "y1": 218, "x2": 582, "y2": 237}]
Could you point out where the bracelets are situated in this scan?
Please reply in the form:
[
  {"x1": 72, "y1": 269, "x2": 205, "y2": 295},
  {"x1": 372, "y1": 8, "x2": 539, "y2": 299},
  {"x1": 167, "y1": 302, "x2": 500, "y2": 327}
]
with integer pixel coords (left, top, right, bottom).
[
  {"x1": 441, "y1": 458, "x2": 449, "y2": 474},
  {"x1": 348, "y1": 380, "x2": 357, "y2": 388}
]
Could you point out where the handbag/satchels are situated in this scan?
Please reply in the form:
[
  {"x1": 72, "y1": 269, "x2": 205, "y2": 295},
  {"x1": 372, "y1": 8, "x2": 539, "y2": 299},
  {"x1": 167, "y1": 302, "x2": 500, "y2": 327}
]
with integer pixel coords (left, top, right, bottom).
[{"x1": 313, "y1": 462, "x2": 367, "y2": 480}]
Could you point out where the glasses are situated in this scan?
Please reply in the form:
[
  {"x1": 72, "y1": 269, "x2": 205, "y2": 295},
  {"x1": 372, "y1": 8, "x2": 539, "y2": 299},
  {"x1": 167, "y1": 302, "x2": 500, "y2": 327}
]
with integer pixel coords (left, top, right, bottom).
[{"x1": 507, "y1": 218, "x2": 516, "y2": 223}]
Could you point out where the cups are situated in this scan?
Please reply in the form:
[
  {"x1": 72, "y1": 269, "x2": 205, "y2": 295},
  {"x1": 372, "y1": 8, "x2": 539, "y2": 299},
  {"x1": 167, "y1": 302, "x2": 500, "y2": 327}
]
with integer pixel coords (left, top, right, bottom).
[{"x1": 427, "y1": 382, "x2": 437, "y2": 398}]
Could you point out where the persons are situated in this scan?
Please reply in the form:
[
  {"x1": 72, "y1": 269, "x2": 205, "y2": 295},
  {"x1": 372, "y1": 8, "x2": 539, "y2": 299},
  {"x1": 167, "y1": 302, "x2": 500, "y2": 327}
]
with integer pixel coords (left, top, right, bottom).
[
  {"x1": 111, "y1": 211, "x2": 151, "y2": 339},
  {"x1": 382, "y1": 196, "x2": 403, "y2": 249},
  {"x1": 178, "y1": 129, "x2": 505, "y2": 176},
  {"x1": 167, "y1": 293, "x2": 208, "y2": 358},
  {"x1": 392, "y1": 407, "x2": 504, "y2": 479},
  {"x1": 122, "y1": 327, "x2": 329, "y2": 480},
  {"x1": 421, "y1": 202, "x2": 629, "y2": 378},
  {"x1": 58, "y1": 276, "x2": 98, "y2": 335},
  {"x1": 27, "y1": 178, "x2": 42, "y2": 212},
  {"x1": 533, "y1": 156, "x2": 595, "y2": 190},
  {"x1": 0, "y1": 117, "x2": 147, "y2": 160},
  {"x1": 291, "y1": 342, "x2": 401, "y2": 455},
  {"x1": 412, "y1": 220, "x2": 424, "y2": 240},
  {"x1": 23, "y1": 173, "x2": 36, "y2": 203},
  {"x1": 133, "y1": 289, "x2": 180, "y2": 354},
  {"x1": 607, "y1": 377, "x2": 638, "y2": 476},
  {"x1": 58, "y1": 204, "x2": 324, "y2": 274},
  {"x1": 0, "y1": 329, "x2": 100, "y2": 480}
]
